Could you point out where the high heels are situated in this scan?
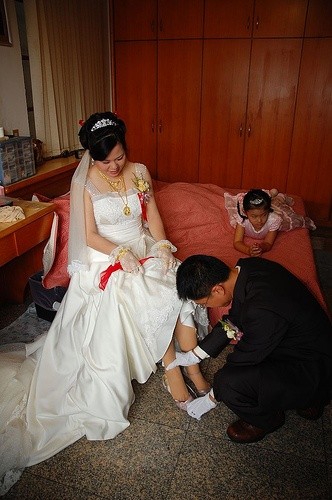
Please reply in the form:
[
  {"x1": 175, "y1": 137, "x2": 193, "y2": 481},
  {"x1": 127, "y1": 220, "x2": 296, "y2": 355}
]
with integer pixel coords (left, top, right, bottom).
[
  {"x1": 162, "y1": 374, "x2": 194, "y2": 412},
  {"x1": 183, "y1": 366, "x2": 210, "y2": 394}
]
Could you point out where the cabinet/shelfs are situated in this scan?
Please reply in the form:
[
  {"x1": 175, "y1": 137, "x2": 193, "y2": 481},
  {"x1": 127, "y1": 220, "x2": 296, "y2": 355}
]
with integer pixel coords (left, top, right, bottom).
[{"x1": 111, "y1": 0, "x2": 332, "y2": 230}]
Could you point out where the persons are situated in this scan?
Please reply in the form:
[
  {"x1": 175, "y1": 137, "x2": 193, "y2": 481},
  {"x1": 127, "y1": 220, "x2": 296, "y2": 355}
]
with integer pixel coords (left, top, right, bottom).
[
  {"x1": 224, "y1": 188, "x2": 317, "y2": 257},
  {"x1": 162, "y1": 255, "x2": 332, "y2": 442},
  {"x1": 0, "y1": 111, "x2": 212, "y2": 496}
]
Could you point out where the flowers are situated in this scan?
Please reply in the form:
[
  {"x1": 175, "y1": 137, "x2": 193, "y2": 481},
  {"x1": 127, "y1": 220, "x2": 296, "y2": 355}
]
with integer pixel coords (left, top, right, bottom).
[
  {"x1": 132, "y1": 171, "x2": 150, "y2": 221},
  {"x1": 218, "y1": 318, "x2": 244, "y2": 345}
]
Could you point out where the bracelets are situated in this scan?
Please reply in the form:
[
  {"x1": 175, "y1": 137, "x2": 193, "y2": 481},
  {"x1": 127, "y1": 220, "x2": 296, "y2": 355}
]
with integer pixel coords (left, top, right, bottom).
[
  {"x1": 191, "y1": 349, "x2": 203, "y2": 361},
  {"x1": 159, "y1": 245, "x2": 171, "y2": 250},
  {"x1": 116, "y1": 248, "x2": 128, "y2": 262}
]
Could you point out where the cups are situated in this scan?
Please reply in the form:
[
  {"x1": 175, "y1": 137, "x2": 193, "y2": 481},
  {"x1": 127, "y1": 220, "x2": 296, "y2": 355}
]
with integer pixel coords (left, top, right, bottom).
[{"x1": 0, "y1": 127, "x2": 4, "y2": 138}]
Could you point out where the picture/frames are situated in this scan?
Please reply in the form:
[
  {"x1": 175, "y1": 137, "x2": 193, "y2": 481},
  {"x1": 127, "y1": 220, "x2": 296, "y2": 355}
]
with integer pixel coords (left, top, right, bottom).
[{"x1": 0, "y1": 0, "x2": 13, "y2": 47}]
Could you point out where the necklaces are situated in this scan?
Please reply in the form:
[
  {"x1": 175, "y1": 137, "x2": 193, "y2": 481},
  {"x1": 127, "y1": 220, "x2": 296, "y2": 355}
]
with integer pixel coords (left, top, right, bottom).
[{"x1": 94, "y1": 165, "x2": 131, "y2": 216}]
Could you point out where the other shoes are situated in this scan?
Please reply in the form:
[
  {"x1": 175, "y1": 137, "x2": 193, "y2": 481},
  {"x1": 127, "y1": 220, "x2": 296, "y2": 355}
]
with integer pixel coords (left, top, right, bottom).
[{"x1": 227, "y1": 414, "x2": 285, "y2": 443}]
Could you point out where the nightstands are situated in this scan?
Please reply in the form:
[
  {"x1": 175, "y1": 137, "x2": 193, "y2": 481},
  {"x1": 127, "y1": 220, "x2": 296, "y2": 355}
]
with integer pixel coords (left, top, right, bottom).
[{"x1": 0, "y1": 157, "x2": 82, "y2": 266}]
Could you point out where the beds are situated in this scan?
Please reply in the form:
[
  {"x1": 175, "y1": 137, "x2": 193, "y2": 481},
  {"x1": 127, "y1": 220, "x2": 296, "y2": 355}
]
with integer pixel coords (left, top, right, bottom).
[{"x1": 31, "y1": 171, "x2": 328, "y2": 331}]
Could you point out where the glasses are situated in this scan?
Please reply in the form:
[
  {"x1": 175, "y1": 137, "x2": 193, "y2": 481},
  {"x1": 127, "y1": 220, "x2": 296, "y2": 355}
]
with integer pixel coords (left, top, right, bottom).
[{"x1": 197, "y1": 294, "x2": 210, "y2": 310}]
